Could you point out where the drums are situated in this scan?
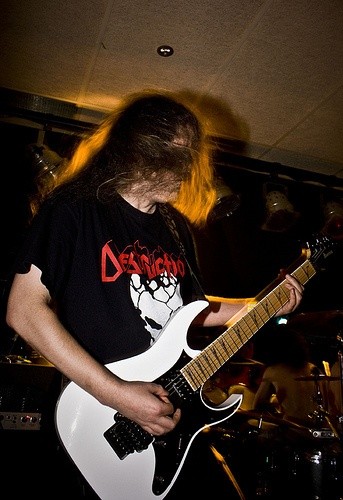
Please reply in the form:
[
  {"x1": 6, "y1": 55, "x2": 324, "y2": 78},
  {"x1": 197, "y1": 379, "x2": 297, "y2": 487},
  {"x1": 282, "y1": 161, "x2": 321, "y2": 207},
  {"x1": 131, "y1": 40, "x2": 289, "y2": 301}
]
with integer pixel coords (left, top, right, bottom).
[{"x1": 291, "y1": 427, "x2": 340, "y2": 482}]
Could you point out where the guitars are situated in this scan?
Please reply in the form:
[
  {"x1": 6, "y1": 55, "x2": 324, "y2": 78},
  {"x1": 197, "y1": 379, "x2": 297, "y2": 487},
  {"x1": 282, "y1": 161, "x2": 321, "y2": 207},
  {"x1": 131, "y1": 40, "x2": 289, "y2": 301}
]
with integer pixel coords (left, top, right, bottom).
[{"x1": 54, "y1": 232, "x2": 335, "y2": 500}]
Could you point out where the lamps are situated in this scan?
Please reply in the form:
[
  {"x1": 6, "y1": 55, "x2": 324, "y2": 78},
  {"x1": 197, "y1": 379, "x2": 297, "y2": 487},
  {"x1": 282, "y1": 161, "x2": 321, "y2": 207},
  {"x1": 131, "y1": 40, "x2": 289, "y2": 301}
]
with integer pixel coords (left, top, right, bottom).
[
  {"x1": 319, "y1": 199, "x2": 343, "y2": 241},
  {"x1": 261, "y1": 182, "x2": 301, "y2": 232},
  {"x1": 29, "y1": 130, "x2": 67, "y2": 180},
  {"x1": 208, "y1": 177, "x2": 240, "y2": 220}
]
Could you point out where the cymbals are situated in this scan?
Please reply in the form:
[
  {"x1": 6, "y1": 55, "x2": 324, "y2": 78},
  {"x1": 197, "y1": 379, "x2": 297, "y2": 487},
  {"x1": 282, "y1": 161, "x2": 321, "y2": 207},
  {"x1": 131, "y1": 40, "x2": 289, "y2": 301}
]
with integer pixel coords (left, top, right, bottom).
[
  {"x1": 274, "y1": 308, "x2": 343, "y2": 338},
  {"x1": 293, "y1": 372, "x2": 341, "y2": 381}
]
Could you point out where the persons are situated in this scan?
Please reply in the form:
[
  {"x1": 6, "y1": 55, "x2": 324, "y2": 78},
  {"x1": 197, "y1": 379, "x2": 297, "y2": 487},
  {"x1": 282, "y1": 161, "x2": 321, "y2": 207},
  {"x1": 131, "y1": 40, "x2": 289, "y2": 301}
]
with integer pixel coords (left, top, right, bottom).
[
  {"x1": 251, "y1": 323, "x2": 328, "y2": 500},
  {"x1": 4, "y1": 87, "x2": 307, "y2": 500}
]
